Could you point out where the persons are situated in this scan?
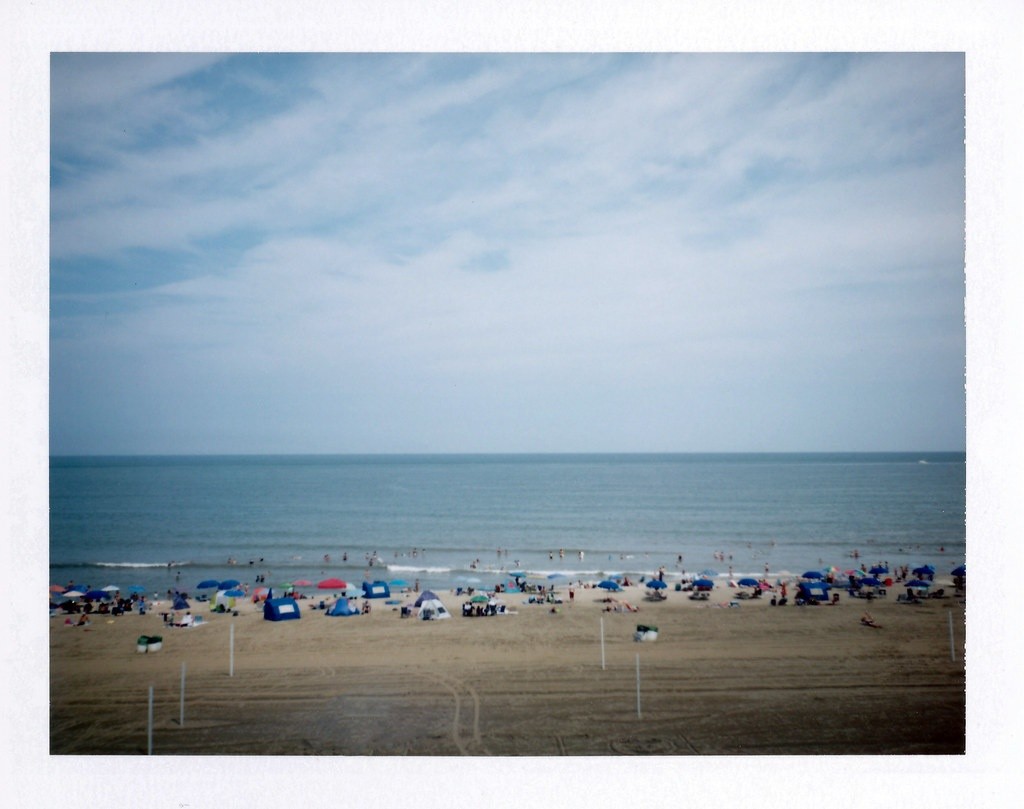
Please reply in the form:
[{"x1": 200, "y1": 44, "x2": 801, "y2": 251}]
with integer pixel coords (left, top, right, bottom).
[{"x1": 68, "y1": 542, "x2": 955, "y2": 629}]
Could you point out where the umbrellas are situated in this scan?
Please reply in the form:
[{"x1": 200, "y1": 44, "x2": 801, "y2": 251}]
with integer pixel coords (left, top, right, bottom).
[
  {"x1": 904, "y1": 579, "x2": 930, "y2": 587},
  {"x1": 692, "y1": 579, "x2": 714, "y2": 586},
  {"x1": 598, "y1": 581, "x2": 620, "y2": 589},
  {"x1": 861, "y1": 578, "x2": 881, "y2": 587},
  {"x1": 646, "y1": 580, "x2": 667, "y2": 589},
  {"x1": 802, "y1": 571, "x2": 823, "y2": 579},
  {"x1": 951, "y1": 565, "x2": 966, "y2": 576},
  {"x1": 912, "y1": 566, "x2": 935, "y2": 576},
  {"x1": 869, "y1": 567, "x2": 889, "y2": 575},
  {"x1": 49, "y1": 584, "x2": 86, "y2": 597},
  {"x1": 317, "y1": 577, "x2": 346, "y2": 589},
  {"x1": 738, "y1": 578, "x2": 759, "y2": 586}
]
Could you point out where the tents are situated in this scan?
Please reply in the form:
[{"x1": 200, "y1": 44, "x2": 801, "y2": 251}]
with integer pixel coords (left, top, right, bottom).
[
  {"x1": 414, "y1": 590, "x2": 440, "y2": 608},
  {"x1": 325, "y1": 597, "x2": 360, "y2": 616},
  {"x1": 340, "y1": 583, "x2": 366, "y2": 598},
  {"x1": 418, "y1": 599, "x2": 451, "y2": 620},
  {"x1": 362, "y1": 580, "x2": 391, "y2": 599},
  {"x1": 264, "y1": 597, "x2": 302, "y2": 620}
]
